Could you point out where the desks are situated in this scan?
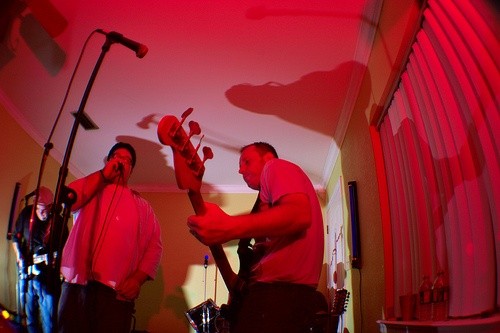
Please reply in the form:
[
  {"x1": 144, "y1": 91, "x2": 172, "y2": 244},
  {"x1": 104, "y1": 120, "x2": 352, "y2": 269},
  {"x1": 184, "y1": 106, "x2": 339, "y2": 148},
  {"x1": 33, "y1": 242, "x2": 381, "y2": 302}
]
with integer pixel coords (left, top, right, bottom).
[{"x1": 376, "y1": 315, "x2": 500, "y2": 333}]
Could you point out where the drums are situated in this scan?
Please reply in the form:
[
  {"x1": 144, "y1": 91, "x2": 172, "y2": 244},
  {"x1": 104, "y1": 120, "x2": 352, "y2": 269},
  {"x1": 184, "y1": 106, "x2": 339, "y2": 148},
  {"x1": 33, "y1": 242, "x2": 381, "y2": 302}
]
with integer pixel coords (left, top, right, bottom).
[{"x1": 184, "y1": 298, "x2": 221, "y2": 333}]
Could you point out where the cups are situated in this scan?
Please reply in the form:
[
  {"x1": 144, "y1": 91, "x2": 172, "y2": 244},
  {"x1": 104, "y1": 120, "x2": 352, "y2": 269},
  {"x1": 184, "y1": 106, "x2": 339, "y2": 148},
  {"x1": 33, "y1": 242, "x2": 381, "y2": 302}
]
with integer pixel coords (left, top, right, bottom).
[{"x1": 399, "y1": 293, "x2": 417, "y2": 320}]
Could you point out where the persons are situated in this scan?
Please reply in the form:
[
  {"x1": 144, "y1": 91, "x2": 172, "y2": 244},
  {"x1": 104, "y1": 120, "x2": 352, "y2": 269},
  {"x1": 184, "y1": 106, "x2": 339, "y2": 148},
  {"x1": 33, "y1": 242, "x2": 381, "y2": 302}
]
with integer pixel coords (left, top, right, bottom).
[
  {"x1": 186, "y1": 141, "x2": 324, "y2": 333},
  {"x1": 54, "y1": 142, "x2": 161, "y2": 333},
  {"x1": 12, "y1": 187, "x2": 70, "y2": 333}
]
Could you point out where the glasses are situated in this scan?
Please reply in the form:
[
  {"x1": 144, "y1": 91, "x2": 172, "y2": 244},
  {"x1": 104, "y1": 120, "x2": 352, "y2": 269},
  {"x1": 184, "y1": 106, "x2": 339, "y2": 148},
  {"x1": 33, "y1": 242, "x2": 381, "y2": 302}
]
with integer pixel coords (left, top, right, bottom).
[{"x1": 113, "y1": 153, "x2": 130, "y2": 163}]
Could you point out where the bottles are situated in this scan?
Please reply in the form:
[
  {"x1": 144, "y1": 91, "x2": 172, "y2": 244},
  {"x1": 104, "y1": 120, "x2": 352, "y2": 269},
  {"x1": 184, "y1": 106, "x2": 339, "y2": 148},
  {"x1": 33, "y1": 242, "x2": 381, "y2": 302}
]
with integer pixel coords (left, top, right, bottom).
[
  {"x1": 419, "y1": 275, "x2": 433, "y2": 321},
  {"x1": 432, "y1": 272, "x2": 449, "y2": 321}
]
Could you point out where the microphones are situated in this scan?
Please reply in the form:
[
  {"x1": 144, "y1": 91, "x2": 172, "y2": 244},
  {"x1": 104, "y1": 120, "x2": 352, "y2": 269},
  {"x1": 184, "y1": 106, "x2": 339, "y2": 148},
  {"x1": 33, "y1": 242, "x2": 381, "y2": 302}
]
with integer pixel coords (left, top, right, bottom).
[
  {"x1": 96, "y1": 29, "x2": 149, "y2": 58},
  {"x1": 117, "y1": 162, "x2": 123, "y2": 170}
]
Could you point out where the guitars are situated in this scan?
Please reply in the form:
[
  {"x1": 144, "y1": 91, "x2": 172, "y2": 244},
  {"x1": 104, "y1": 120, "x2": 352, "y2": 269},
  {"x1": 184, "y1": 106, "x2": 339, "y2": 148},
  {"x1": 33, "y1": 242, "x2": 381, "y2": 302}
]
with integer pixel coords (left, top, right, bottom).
[
  {"x1": 18, "y1": 246, "x2": 61, "y2": 280},
  {"x1": 329, "y1": 288, "x2": 350, "y2": 333},
  {"x1": 156, "y1": 106, "x2": 240, "y2": 304}
]
